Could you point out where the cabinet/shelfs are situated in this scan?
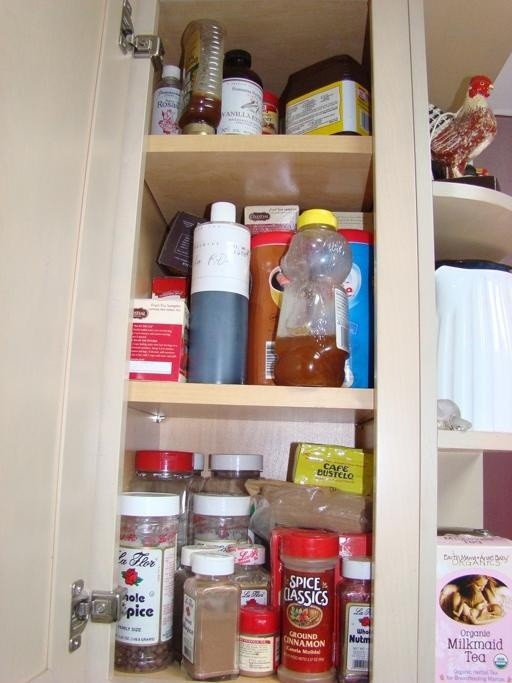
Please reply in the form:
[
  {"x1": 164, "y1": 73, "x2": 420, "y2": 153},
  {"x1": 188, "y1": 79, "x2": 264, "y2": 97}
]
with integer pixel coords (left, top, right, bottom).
[{"x1": 3, "y1": 2, "x2": 512, "y2": 683}]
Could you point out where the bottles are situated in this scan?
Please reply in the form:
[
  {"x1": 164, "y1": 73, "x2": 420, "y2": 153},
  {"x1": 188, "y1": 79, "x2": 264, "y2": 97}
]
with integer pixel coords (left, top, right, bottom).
[
  {"x1": 114, "y1": 491, "x2": 180, "y2": 674},
  {"x1": 182, "y1": 549, "x2": 240, "y2": 682},
  {"x1": 187, "y1": 493, "x2": 252, "y2": 552},
  {"x1": 192, "y1": 454, "x2": 207, "y2": 494},
  {"x1": 150, "y1": 64, "x2": 184, "y2": 134},
  {"x1": 240, "y1": 545, "x2": 270, "y2": 607},
  {"x1": 217, "y1": 48, "x2": 263, "y2": 135},
  {"x1": 248, "y1": 232, "x2": 292, "y2": 386},
  {"x1": 182, "y1": 19, "x2": 224, "y2": 134},
  {"x1": 338, "y1": 228, "x2": 373, "y2": 388},
  {"x1": 262, "y1": 90, "x2": 280, "y2": 134},
  {"x1": 239, "y1": 603, "x2": 280, "y2": 676},
  {"x1": 274, "y1": 209, "x2": 352, "y2": 386},
  {"x1": 206, "y1": 454, "x2": 264, "y2": 493},
  {"x1": 280, "y1": 525, "x2": 338, "y2": 683},
  {"x1": 187, "y1": 202, "x2": 252, "y2": 384},
  {"x1": 336, "y1": 556, "x2": 371, "y2": 683},
  {"x1": 134, "y1": 449, "x2": 193, "y2": 663}
]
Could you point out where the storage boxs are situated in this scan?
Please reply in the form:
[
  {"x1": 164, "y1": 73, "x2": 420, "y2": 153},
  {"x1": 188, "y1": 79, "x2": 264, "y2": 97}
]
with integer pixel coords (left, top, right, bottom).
[{"x1": 435, "y1": 536, "x2": 512, "y2": 683}]
